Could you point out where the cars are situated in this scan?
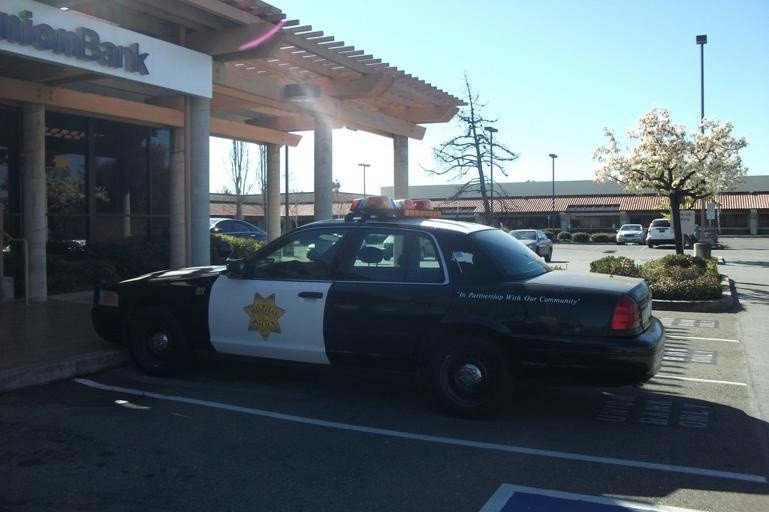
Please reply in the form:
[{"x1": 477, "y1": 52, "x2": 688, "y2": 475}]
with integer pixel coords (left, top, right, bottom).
[
  {"x1": 87, "y1": 192, "x2": 674, "y2": 416},
  {"x1": 209, "y1": 214, "x2": 267, "y2": 264},
  {"x1": 616, "y1": 221, "x2": 646, "y2": 246},
  {"x1": 506, "y1": 228, "x2": 557, "y2": 263}
]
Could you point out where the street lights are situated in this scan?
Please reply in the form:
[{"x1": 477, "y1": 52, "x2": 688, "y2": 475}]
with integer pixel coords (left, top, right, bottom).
[
  {"x1": 356, "y1": 162, "x2": 373, "y2": 197},
  {"x1": 484, "y1": 125, "x2": 497, "y2": 219},
  {"x1": 693, "y1": 29, "x2": 714, "y2": 259},
  {"x1": 549, "y1": 152, "x2": 559, "y2": 230}
]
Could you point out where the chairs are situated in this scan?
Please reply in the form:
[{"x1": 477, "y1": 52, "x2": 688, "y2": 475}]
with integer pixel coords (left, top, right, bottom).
[{"x1": 358, "y1": 246, "x2": 384, "y2": 280}]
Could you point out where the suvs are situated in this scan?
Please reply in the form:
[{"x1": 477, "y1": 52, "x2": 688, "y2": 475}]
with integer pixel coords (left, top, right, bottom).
[{"x1": 645, "y1": 218, "x2": 676, "y2": 248}]
